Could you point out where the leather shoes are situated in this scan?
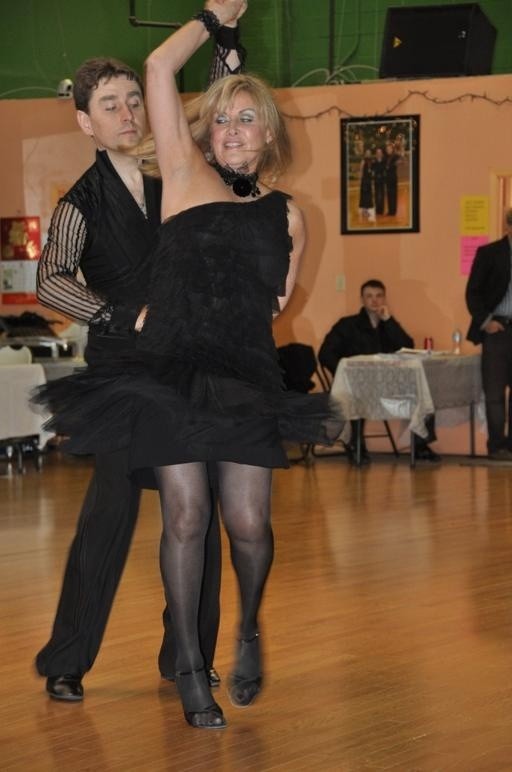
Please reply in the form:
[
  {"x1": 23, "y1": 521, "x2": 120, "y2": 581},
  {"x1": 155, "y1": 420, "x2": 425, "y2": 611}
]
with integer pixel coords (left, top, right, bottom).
[
  {"x1": 45, "y1": 671, "x2": 87, "y2": 702},
  {"x1": 158, "y1": 656, "x2": 223, "y2": 688}
]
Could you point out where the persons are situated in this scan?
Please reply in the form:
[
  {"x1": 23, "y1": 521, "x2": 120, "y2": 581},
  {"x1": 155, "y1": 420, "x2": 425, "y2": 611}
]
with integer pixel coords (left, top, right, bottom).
[
  {"x1": 36, "y1": 0, "x2": 249, "y2": 703},
  {"x1": 464, "y1": 207, "x2": 512, "y2": 460},
  {"x1": 139, "y1": 0, "x2": 306, "y2": 730},
  {"x1": 318, "y1": 280, "x2": 441, "y2": 468},
  {"x1": 348, "y1": 122, "x2": 409, "y2": 224}
]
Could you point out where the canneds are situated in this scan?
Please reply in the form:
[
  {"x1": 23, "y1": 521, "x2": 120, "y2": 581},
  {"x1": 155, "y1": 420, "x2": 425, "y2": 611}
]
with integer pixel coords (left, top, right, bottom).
[{"x1": 424, "y1": 337, "x2": 433, "y2": 350}]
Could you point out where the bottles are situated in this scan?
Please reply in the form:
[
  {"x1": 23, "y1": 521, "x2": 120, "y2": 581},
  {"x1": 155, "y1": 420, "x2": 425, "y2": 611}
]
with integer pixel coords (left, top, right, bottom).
[{"x1": 452, "y1": 328, "x2": 463, "y2": 356}]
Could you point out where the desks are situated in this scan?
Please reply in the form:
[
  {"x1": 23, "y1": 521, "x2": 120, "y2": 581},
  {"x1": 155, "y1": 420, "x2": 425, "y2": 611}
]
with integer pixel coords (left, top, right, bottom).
[{"x1": 329, "y1": 351, "x2": 481, "y2": 468}]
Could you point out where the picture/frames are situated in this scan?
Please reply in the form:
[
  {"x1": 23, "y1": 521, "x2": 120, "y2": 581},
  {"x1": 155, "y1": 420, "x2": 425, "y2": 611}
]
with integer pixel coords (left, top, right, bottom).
[{"x1": 340, "y1": 114, "x2": 420, "y2": 235}]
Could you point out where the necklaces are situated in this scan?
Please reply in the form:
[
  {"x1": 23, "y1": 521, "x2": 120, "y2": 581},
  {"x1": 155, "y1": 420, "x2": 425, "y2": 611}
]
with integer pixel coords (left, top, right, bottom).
[{"x1": 215, "y1": 164, "x2": 262, "y2": 198}]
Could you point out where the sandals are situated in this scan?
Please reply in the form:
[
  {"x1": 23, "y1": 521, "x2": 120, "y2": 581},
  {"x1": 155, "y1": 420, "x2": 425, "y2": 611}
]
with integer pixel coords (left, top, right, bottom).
[
  {"x1": 229, "y1": 631, "x2": 267, "y2": 709},
  {"x1": 174, "y1": 660, "x2": 228, "y2": 731}
]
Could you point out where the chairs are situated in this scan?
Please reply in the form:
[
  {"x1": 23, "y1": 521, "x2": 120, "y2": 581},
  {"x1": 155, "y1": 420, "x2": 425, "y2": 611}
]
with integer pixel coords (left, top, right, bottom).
[
  {"x1": 279, "y1": 340, "x2": 399, "y2": 466},
  {"x1": 0, "y1": 332, "x2": 91, "y2": 477}
]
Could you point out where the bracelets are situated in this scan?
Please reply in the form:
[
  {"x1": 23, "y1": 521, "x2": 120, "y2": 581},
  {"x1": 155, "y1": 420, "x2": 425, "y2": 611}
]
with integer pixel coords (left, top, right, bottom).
[{"x1": 193, "y1": 8, "x2": 222, "y2": 40}]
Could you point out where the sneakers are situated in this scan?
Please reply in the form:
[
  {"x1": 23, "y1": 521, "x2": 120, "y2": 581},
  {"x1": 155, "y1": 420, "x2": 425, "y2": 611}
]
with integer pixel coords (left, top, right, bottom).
[
  {"x1": 486, "y1": 444, "x2": 512, "y2": 460},
  {"x1": 412, "y1": 444, "x2": 443, "y2": 461},
  {"x1": 350, "y1": 436, "x2": 371, "y2": 461}
]
